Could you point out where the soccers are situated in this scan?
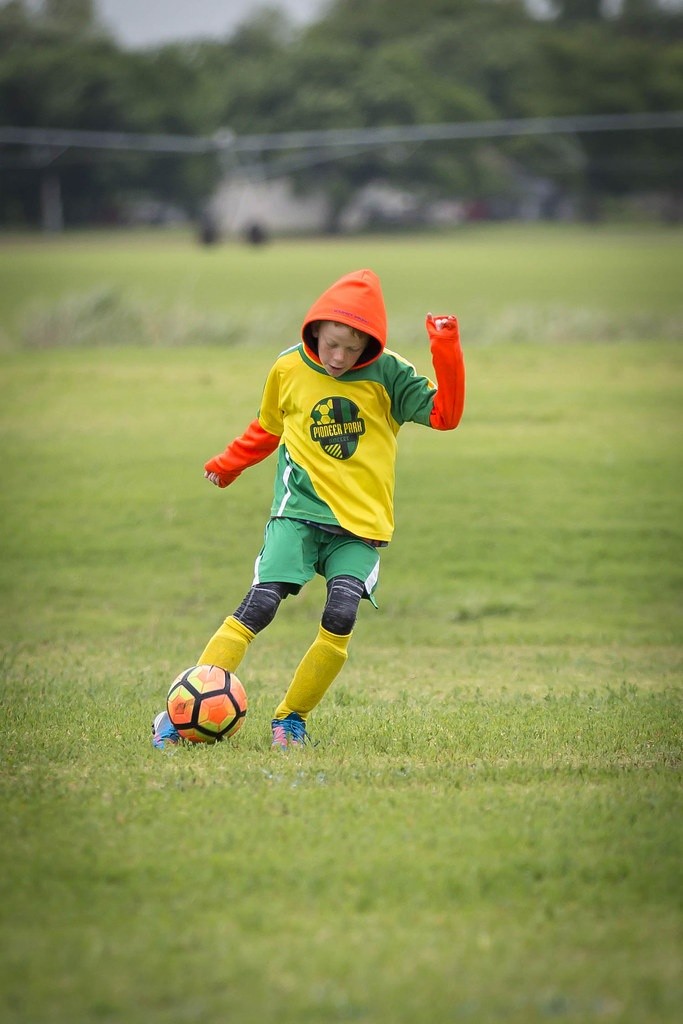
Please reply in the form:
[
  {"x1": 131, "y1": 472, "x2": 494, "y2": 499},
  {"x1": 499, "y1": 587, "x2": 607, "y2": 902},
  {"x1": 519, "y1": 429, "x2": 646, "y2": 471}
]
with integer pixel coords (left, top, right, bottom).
[{"x1": 166, "y1": 664, "x2": 249, "y2": 745}]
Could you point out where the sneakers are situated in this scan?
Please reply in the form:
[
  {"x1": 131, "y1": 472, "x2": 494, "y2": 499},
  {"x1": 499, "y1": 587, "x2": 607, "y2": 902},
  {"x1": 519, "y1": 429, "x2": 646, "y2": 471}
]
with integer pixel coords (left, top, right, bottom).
[
  {"x1": 270, "y1": 710, "x2": 312, "y2": 748},
  {"x1": 152, "y1": 710, "x2": 182, "y2": 749}
]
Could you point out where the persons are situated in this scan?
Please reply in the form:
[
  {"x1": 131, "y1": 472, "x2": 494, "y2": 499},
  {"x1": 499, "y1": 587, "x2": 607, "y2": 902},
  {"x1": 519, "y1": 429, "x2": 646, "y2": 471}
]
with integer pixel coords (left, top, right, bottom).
[{"x1": 151, "y1": 269, "x2": 464, "y2": 750}]
